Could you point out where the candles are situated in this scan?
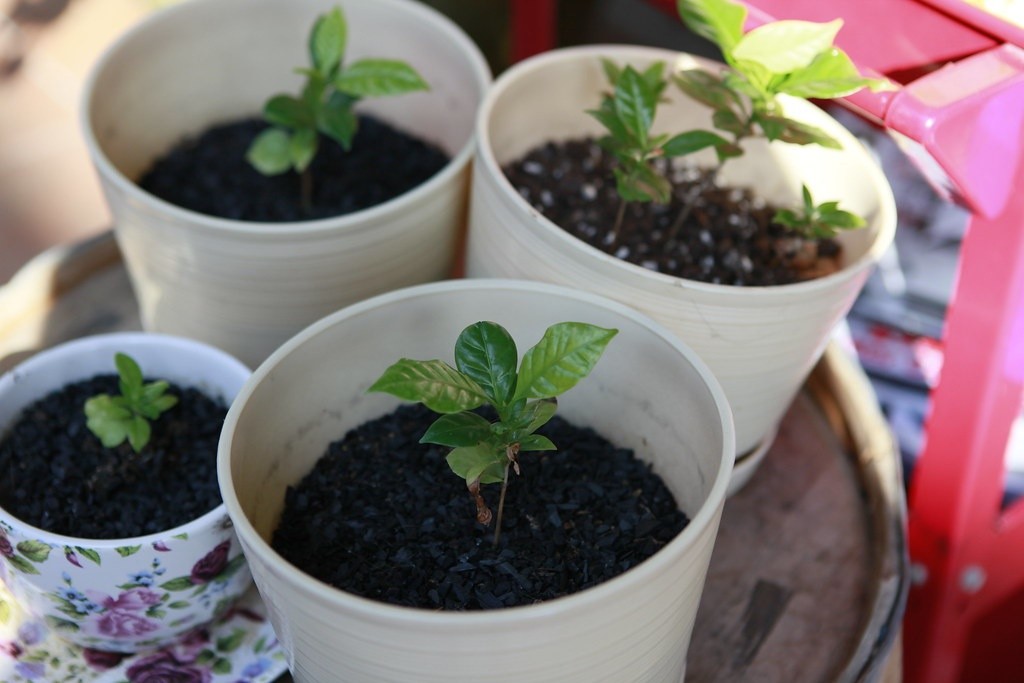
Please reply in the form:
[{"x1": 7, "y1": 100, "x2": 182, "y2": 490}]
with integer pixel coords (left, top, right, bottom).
[{"x1": 0, "y1": 330, "x2": 253, "y2": 654}]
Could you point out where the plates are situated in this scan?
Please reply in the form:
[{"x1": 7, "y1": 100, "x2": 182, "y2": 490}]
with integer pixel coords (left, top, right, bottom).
[{"x1": 0, "y1": 576, "x2": 288, "y2": 683}]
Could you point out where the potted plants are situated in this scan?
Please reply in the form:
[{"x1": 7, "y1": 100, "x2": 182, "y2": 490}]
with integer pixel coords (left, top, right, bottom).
[
  {"x1": 465, "y1": 0, "x2": 899, "y2": 465},
  {"x1": 216, "y1": 278, "x2": 738, "y2": 683},
  {"x1": 78, "y1": 0, "x2": 492, "y2": 373}
]
